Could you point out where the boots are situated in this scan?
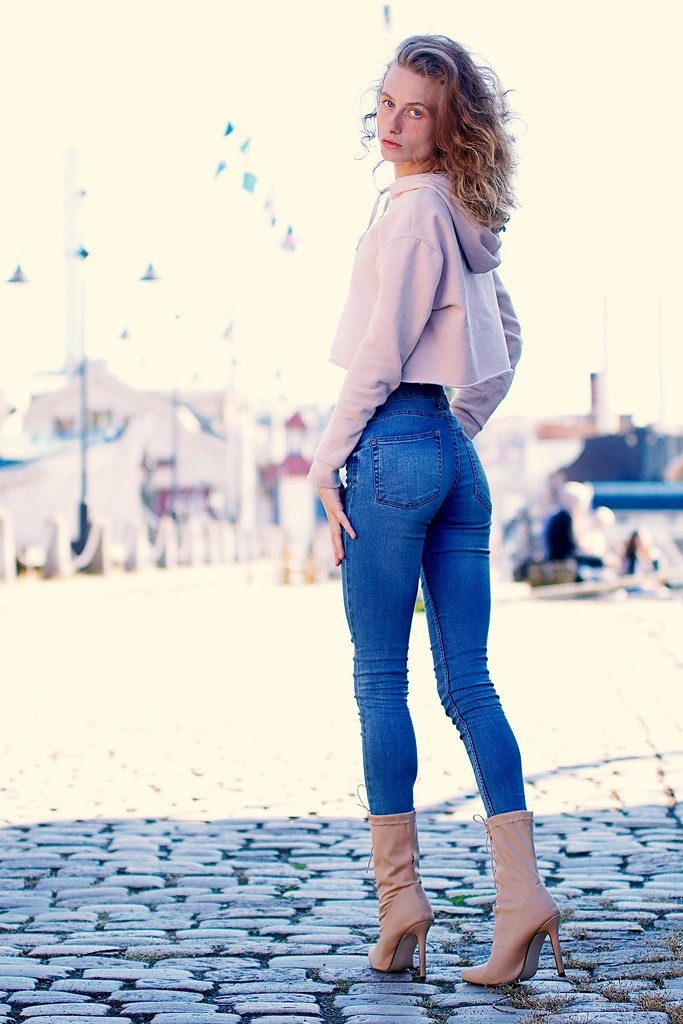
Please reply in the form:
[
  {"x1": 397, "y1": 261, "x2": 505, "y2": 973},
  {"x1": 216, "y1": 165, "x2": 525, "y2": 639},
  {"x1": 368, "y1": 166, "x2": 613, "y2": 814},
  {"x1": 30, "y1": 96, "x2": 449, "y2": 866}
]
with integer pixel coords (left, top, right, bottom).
[
  {"x1": 367, "y1": 809, "x2": 436, "y2": 980},
  {"x1": 459, "y1": 810, "x2": 566, "y2": 988}
]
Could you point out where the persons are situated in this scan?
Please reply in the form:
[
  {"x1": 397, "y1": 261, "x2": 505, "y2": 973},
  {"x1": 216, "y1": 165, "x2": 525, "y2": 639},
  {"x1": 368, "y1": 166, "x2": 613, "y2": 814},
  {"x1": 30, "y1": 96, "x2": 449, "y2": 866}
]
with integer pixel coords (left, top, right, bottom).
[{"x1": 306, "y1": 33, "x2": 557, "y2": 983}]
